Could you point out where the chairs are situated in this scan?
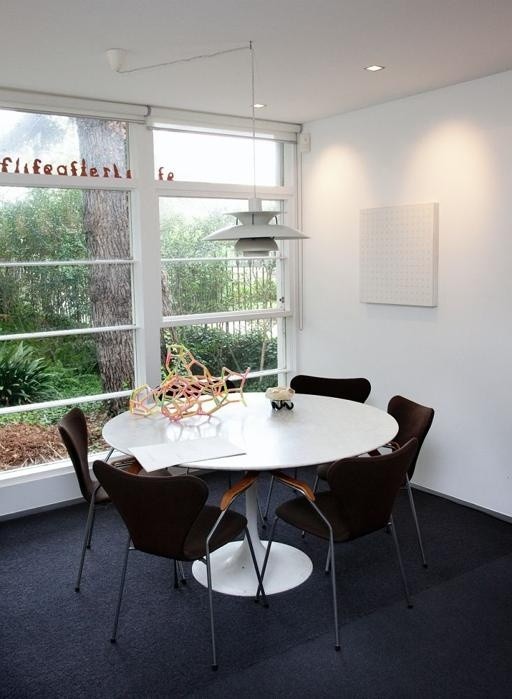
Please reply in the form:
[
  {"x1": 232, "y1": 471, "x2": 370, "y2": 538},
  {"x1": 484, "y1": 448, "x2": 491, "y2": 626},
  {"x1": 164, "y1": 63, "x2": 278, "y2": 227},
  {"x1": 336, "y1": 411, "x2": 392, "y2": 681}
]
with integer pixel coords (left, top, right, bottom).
[
  {"x1": 301, "y1": 395, "x2": 435, "y2": 574},
  {"x1": 255, "y1": 437, "x2": 419, "y2": 651},
  {"x1": 263, "y1": 375, "x2": 372, "y2": 519},
  {"x1": 57, "y1": 406, "x2": 187, "y2": 592},
  {"x1": 93, "y1": 460, "x2": 268, "y2": 672}
]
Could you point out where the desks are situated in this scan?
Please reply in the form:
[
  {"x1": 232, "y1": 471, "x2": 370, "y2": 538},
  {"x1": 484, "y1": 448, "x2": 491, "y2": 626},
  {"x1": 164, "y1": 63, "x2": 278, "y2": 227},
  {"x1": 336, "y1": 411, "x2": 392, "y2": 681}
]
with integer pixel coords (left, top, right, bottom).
[{"x1": 102, "y1": 391, "x2": 399, "y2": 598}]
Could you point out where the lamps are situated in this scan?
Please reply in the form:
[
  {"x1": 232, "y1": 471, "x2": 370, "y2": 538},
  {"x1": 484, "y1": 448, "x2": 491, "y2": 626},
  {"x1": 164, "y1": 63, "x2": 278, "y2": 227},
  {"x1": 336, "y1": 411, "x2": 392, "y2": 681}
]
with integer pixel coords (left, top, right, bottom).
[{"x1": 106, "y1": 47, "x2": 309, "y2": 257}]
